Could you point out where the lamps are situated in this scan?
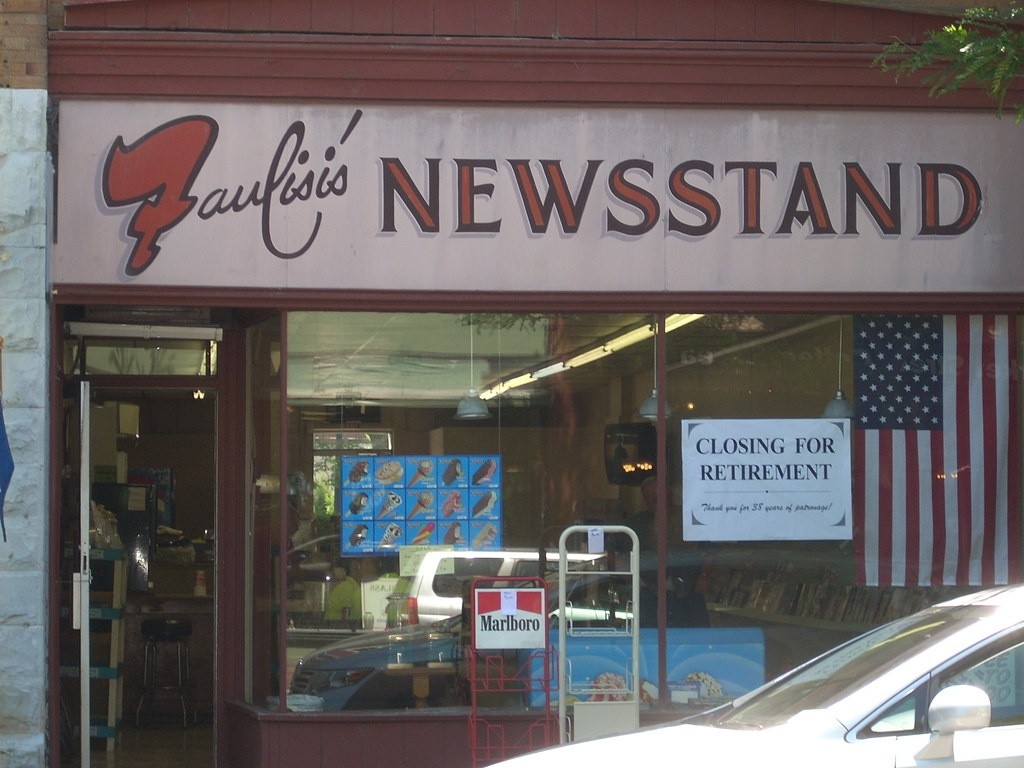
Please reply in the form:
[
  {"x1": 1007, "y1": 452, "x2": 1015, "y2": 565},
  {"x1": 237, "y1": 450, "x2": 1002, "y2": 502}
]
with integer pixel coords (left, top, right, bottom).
[
  {"x1": 450, "y1": 313, "x2": 493, "y2": 423},
  {"x1": 638, "y1": 312, "x2": 673, "y2": 420},
  {"x1": 819, "y1": 313, "x2": 854, "y2": 418}
]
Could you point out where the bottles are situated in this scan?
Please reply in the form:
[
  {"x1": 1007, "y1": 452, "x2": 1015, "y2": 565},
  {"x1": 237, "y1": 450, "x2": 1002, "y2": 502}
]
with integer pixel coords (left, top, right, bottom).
[{"x1": 194, "y1": 570, "x2": 206, "y2": 595}]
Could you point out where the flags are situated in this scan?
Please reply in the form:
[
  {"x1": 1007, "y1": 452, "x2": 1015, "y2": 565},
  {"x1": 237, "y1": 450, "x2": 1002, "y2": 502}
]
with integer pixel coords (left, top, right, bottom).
[{"x1": 853, "y1": 314, "x2": 1022, "y2": 586}]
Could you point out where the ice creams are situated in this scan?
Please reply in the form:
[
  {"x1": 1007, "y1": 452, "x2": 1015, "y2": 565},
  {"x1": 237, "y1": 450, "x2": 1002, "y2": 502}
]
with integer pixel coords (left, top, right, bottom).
[{"x1": 349, "y1": 459, "x2": 496, "y2": 550}]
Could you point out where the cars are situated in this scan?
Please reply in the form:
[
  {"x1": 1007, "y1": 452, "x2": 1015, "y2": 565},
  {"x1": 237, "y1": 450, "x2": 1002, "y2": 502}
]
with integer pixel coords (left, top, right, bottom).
[
  {"x1": 288, "y1": 532, "x2": 401, "y2": 628},
  {"x1": 485, "y1": 582, "x2": 1024, "y2": 768},
  {"x1": 286, "y1": 540, "x2": 966, "y2": 708}
]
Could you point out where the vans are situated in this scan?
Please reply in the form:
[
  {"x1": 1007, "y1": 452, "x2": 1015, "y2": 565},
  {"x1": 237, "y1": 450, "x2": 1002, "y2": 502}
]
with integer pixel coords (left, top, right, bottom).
[{"x1": 385, "y1": 548, "x2": 608, "y2": 629}]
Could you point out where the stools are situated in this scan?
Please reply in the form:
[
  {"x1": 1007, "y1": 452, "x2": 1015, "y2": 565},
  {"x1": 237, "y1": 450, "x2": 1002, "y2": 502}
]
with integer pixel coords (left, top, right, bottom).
[{"x1": 134, "y1": 616, "x2": 198, "y2": 729}]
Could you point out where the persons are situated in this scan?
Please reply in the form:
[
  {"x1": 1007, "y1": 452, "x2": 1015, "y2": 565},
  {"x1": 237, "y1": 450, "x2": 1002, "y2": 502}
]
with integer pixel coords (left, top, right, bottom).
[
  {"x1": 324, "y1": 554, "x2": 414, "y2": 620},
  {"x1": 630, "y1": 476, "x2": 698, "y2": 549}
]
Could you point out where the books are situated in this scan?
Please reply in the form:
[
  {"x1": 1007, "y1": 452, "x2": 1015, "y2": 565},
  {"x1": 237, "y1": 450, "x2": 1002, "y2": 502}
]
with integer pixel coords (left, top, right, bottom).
[{"x1": 719, "y1": 571, "x2": 924, "y2": 623}]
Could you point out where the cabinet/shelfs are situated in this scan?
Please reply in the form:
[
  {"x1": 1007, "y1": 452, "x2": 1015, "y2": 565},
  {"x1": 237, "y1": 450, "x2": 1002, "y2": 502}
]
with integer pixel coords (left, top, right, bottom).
[
  {"x1": 467, "y1": 524, "x2": 641, "y2": 767},
  {"x1": 63, "y1": 540, "x2": 128, "y2": 751}
]
